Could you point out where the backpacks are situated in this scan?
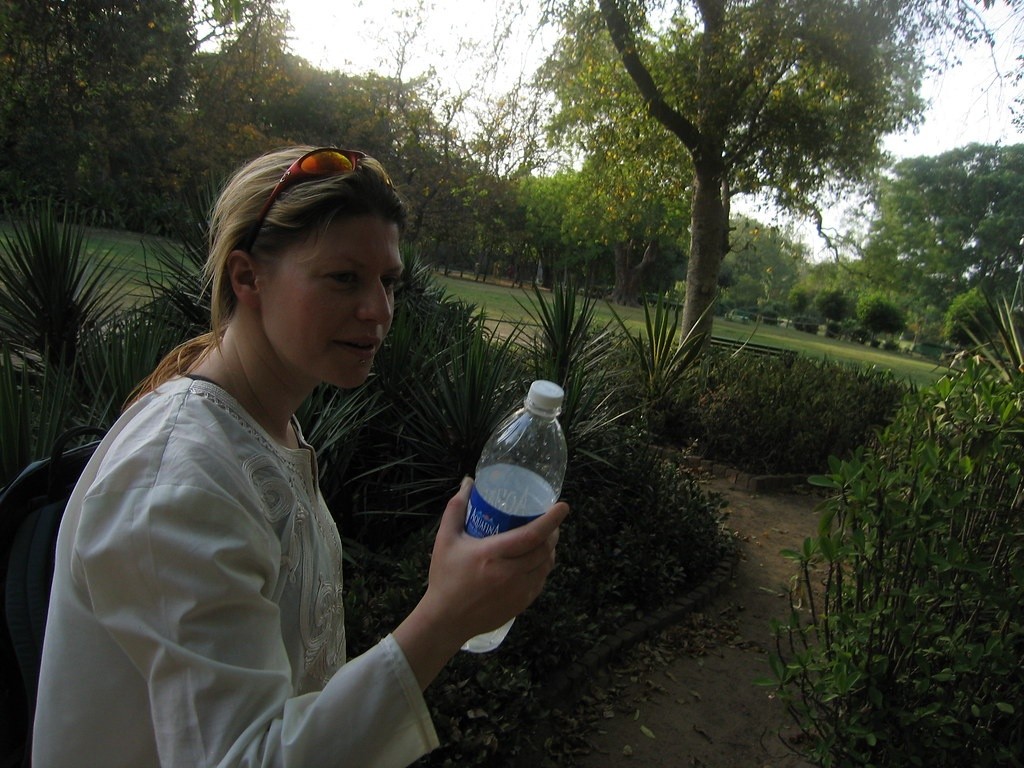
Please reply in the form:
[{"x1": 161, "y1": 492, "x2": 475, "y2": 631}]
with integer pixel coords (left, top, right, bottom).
[{"x1": 0, "y1": 421, "x2": 111, "y2": 768}]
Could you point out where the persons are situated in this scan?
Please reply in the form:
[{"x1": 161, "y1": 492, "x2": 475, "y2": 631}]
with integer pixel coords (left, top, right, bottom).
[{"x1": 32, "y1": 146, "x2": 571, "y2": 768}]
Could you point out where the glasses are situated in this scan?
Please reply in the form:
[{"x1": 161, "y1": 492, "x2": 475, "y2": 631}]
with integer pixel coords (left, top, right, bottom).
[{"x1": 242, "y1": 148, "x2": 369, "y2": 251}]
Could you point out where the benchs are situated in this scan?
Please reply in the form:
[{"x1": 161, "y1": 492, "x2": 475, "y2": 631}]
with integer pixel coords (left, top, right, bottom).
[{"x1": 706, "y1": 335, "x2": 798, "y2": 367}]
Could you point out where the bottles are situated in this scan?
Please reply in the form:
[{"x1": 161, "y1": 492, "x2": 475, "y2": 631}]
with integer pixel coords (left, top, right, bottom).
[{"x1": 461, "y1": 380, "x2": 566, "y2": 653}]
